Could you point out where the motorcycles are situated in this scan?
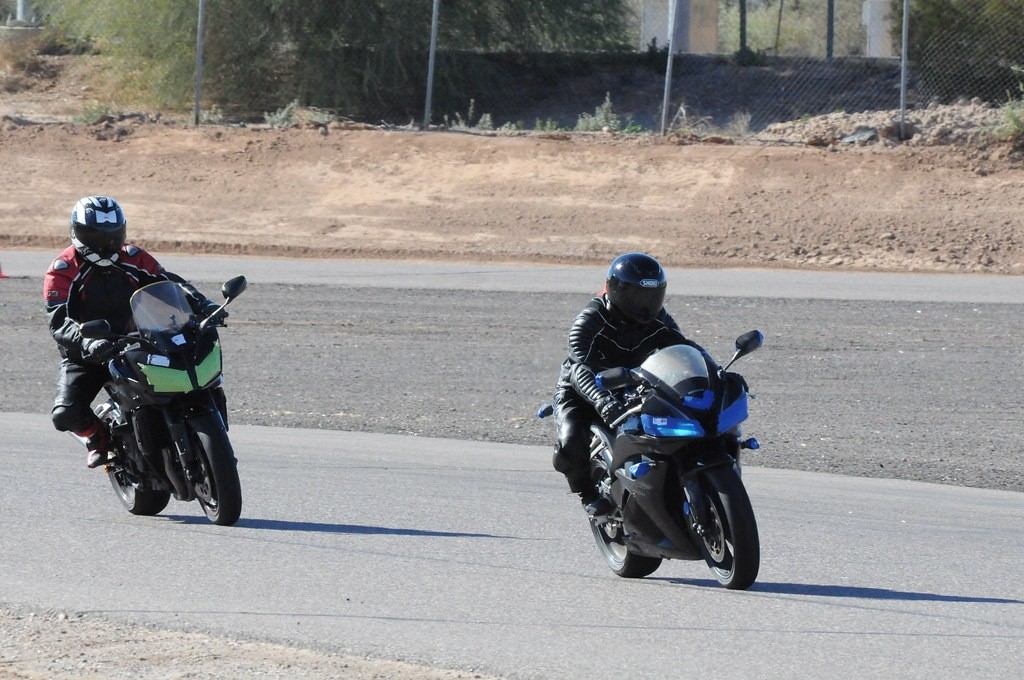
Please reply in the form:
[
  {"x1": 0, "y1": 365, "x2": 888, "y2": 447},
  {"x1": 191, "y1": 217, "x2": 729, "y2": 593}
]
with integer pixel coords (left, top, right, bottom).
[
  {"x1": 77, "y1": 275, "x2": 247, "y2": 526},
  {"x1": 537, "y1": 329, "x2": 763, "y2": 590}
]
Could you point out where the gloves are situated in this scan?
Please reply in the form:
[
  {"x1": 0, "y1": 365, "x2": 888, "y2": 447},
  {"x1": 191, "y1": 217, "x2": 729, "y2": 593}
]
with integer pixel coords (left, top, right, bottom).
[
  {"x1": 722, "y1": 371, "x2": 751, "y2": 393},
  {"x1": 200, "y1": 303, "x2": 228, "y2": 326},
  {"x1": 596, "y1": 400, "x2": 629, "y2": 426},
  {"x1": 86, "y1": 338, "x2": 115, "y2": 359}
]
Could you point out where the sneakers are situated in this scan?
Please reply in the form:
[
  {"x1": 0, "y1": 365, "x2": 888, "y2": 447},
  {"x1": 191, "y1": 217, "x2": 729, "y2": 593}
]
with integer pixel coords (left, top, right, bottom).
[
  {"x1": 580, "y1": 488, "x2": 613, "y2": 516},
  {"x1": 84, "y1": 421, "x2": 111, "y2": 469}
]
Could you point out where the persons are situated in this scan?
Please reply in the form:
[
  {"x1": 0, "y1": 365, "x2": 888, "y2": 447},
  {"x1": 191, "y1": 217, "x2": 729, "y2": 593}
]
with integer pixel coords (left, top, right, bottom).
[
  {"x1": 550, "y1": 252, "x2": 686, "y2": 519},
  {"x1": 41, "y1": 196, "x2": 230, "y2": 470}
]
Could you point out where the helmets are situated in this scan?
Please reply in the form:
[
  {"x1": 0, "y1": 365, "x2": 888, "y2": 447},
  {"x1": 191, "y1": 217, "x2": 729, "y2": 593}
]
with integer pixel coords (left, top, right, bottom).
[
  {"x1": 69, "y1": 196, "x2": 128, "y2": 268},
  {"x1": 605, "y1": 253, "x2": 669, "y2": 318}
]
